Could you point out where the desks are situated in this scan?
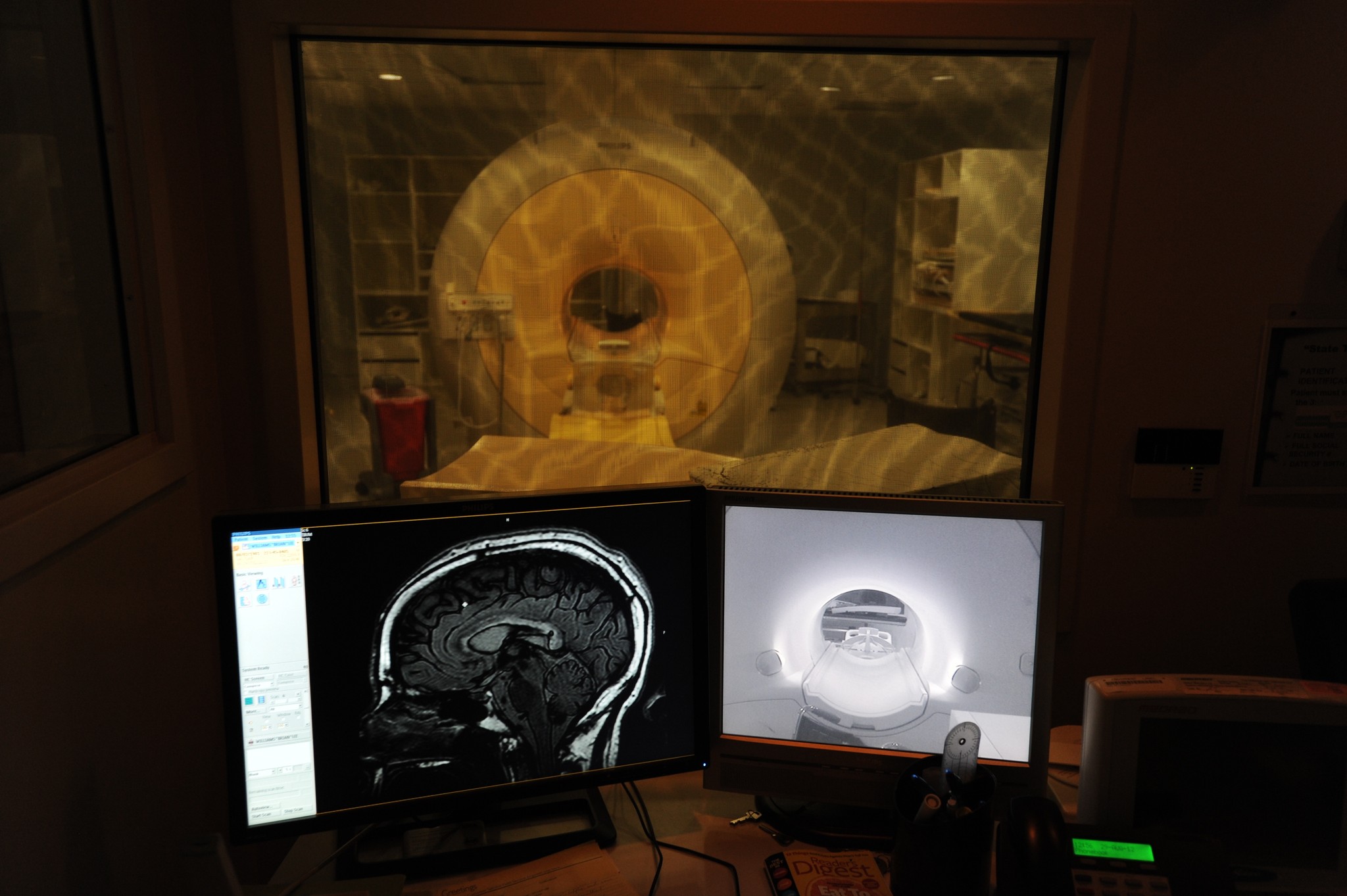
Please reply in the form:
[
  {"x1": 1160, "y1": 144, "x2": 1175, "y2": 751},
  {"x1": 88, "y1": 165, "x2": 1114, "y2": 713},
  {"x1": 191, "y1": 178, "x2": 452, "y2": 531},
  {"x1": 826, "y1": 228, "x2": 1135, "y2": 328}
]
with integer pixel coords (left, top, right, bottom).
[{"x1": 248, "y1": 758, "x2": 1345, "y2": 895}]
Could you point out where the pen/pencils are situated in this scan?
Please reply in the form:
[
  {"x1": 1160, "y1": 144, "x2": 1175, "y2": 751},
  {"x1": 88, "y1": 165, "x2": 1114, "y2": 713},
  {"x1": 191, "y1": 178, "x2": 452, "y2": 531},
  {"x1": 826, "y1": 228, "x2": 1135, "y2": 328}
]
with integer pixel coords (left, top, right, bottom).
[{"x1": 909, "y1": 769, "x2": 987, "y2": 823}]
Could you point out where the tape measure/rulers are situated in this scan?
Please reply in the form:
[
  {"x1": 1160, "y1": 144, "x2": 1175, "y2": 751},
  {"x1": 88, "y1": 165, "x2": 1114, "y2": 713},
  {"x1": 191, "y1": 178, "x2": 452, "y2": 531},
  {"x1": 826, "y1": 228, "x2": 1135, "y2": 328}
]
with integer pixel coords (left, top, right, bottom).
[{"x1": 943, "y1": 722, "x2": 980, "y2": 782}]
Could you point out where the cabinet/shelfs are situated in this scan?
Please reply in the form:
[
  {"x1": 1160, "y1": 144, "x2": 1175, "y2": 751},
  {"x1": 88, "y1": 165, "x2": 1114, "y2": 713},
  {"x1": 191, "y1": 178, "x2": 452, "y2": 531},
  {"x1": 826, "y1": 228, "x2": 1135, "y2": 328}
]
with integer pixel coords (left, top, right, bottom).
[
  {"x1": 343, "y1": 152, "x2": 498, "y2": 405},
  {"x1": 885, "y1": 149, "x2": 1050, "y2": 413}
]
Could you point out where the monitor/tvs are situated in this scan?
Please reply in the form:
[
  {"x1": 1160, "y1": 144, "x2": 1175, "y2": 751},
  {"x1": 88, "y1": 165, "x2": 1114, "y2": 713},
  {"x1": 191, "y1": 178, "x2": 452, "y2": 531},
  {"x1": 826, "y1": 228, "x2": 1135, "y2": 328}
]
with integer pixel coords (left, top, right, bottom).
[
  {"x1": 703, "y1": 486, "x2": 1064, "y2": 853},
  {"x1": 210, "y1": 480, "x2": 715, "y2": 882}
]
